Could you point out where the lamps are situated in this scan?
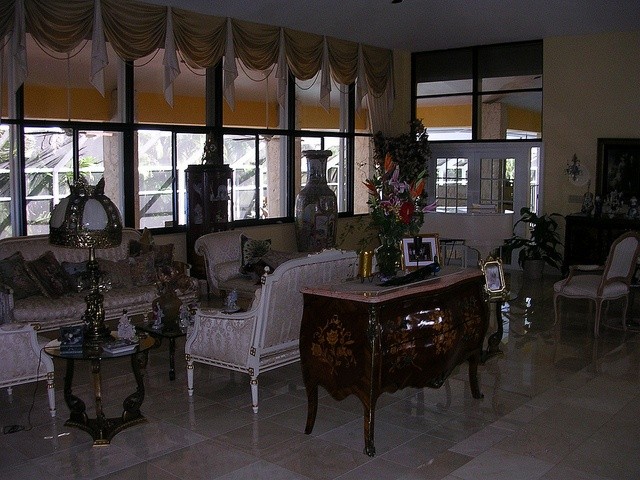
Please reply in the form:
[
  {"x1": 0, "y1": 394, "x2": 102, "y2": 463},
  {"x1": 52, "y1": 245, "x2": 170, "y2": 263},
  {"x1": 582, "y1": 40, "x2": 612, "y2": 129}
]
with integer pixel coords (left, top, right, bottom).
[
  {"x1": 47, "y1": 176, "x2": 124, "y2": 347},
  {"x1": 564, "y1": 152, "x2": 584, "y2": 182}
]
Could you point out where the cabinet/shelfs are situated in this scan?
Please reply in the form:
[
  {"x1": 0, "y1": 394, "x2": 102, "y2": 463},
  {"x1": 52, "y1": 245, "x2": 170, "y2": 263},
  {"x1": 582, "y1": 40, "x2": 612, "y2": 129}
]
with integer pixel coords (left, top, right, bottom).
[
  {"x1": 189, "y1": 165, "x2": 232, "y2": 279},
  {"x1": 565, "y1": 218, "x2": 639, "y2": 264}
]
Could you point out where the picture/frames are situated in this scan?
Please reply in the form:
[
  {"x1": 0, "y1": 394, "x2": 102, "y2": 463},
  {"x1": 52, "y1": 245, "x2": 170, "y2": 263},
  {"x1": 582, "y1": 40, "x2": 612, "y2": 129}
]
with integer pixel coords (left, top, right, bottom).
[
  {"x1": 483, "y1": 260, "x2": 506, "y2": 296},
  {"x1": 401, "y1": 233, "x2": 441, "y2": 270},
  {"x1": 594, "y1": 138, "x2": 640, "y2": 214}
]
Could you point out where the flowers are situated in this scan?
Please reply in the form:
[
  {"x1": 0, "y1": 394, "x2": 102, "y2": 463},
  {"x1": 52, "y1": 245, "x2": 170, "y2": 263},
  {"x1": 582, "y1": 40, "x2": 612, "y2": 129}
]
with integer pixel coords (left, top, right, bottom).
[{"x1": 361, "y1": 118, "x2": 433, "y2": 241}]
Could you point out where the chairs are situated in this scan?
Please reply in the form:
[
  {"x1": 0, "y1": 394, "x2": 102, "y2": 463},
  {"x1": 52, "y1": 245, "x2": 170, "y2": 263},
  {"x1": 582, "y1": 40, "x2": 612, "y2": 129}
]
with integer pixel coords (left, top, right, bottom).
[{"x1": 552, "y1": 231, "x2": 639, "y2": 337}]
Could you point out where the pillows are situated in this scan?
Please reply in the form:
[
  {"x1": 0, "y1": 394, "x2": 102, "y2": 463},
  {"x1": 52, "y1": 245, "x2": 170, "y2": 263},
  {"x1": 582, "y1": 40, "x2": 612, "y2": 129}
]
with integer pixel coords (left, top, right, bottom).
[
  {"x1": 95, "y1": 257, "x2": 132, "y2": 291},
  {"x1": 236, "y1": 233, "x2": 274, "y2": 274},
  {"x1": 61, "y1": 261, "x2": 96, "y2": 292},
  {"x1": 0, "y1": 251, "x2": 53, "y2": 302},
  {"x1": 121, "y1": 249, "x2": 157, "y2": 287},
  {"x1": 128, "y1": 239, "x2": 173, "y2": 279},
  {"x1": 26, "y1": 250, "x2": 74, "y2": 294}
]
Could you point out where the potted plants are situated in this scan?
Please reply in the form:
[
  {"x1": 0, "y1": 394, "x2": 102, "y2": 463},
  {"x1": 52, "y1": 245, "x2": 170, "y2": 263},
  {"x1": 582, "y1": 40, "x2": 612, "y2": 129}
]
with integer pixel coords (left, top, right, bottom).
[{"x1": 488, "y1": 207, "x2": 565, "y2": 279}]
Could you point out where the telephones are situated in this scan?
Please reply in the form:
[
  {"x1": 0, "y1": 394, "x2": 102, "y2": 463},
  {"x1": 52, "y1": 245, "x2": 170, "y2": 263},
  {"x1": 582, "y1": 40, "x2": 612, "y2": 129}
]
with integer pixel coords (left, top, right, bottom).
[{"x1": 58, "y1": 324, "x2": 86, "y2": 355}]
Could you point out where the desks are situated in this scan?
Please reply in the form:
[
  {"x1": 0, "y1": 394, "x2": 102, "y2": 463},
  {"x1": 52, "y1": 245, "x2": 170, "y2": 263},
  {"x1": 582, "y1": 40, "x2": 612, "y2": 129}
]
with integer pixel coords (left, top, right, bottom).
[{"x1": 298, "y1": 265, "x2": 490, "y2": 458}]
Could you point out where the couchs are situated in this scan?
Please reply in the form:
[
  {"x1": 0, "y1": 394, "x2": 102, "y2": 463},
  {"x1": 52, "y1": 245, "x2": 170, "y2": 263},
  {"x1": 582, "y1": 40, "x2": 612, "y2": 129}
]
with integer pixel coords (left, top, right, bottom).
[
  {"x1": 0, "y1": 227, "x2": 202, "y2": 329},
  {"x1": 1, "y1": 322, "x2": 57, "y2": 418},
  {"x1": 193, "y1": 215, "x2": 394, "y2": 302},
  {"x1": 183, "y1": 248, "x2": 390, "y2": 413}
]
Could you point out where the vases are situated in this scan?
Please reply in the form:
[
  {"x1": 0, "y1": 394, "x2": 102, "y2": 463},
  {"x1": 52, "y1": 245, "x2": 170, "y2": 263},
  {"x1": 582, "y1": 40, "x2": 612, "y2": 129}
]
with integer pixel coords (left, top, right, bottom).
[
  {"x1": 295, "y1": 150, "x2": 338, "y2": 253},
  {"x1": 374, "y1": 234, "x2": 402, "y2": 282}
]
